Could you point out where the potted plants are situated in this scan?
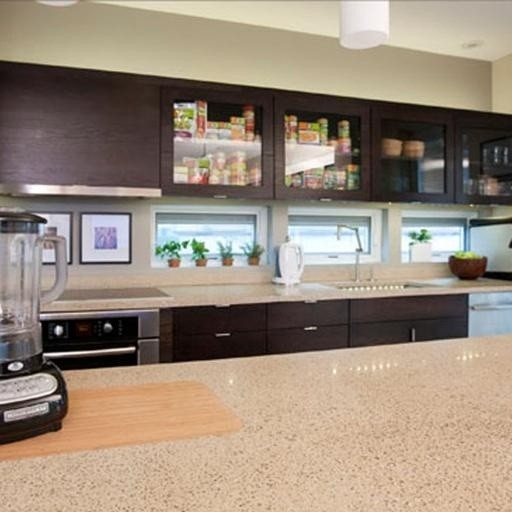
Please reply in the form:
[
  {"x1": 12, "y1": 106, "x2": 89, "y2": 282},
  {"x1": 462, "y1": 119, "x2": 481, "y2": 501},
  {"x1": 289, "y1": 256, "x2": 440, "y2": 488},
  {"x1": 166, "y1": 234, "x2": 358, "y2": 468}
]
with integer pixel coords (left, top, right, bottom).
[
  {"x1": 191, "y1": 237, "x2": 210, "y2": 266},
  {"x1": 155, "y1": 237, "x2": 189, "y2": 268},
  {"x1": 217, "y1": 239, "x2": 235, "y2": 266},
  {"x1": 240, "y1": 240, "x2": 266, "y2": 267},
  {"x1": 407, "y1": 228, "x2": 433, "y2": 264}
]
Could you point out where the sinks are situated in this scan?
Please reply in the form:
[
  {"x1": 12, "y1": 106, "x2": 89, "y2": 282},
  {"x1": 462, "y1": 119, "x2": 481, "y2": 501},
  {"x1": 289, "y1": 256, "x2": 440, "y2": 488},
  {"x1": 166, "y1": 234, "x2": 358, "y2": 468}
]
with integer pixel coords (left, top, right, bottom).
[{"x1": 330, "y1": 282, "x2": 438, "y2": 293}]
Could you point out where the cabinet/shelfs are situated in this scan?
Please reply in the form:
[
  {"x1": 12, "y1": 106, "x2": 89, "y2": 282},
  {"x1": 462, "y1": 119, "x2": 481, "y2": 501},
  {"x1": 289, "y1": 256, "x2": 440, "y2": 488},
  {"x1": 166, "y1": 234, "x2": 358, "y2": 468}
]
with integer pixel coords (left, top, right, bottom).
[
  {"x1": 174, "y1": 303, "x2": 267, "y2": 363},
  {"x1": 267, "y1": 299, "x2": 349, "y2": 355},
  {"x1": 349, "y1": 294, "x2": 469, "y2": 348},
  {"x1": 373, "y1": 99, "x2": 511, "y2": 206},
  {"x1": 469, "y1": 289, "x2": 512, "y2": 338},
  {"x1": 161, "y1": 83, "x2": 373, "y2": 202},
  {"x1": 0, "y1": 60, "x2": 161, "y2": 202}
]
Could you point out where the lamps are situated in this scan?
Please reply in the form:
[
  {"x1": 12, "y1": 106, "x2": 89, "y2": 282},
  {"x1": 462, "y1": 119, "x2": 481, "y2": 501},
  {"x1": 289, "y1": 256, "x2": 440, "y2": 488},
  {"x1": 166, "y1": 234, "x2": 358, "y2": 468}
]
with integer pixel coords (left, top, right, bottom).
[{"x1": 340, "y1": 0, "x2": 391, "y2": 49}]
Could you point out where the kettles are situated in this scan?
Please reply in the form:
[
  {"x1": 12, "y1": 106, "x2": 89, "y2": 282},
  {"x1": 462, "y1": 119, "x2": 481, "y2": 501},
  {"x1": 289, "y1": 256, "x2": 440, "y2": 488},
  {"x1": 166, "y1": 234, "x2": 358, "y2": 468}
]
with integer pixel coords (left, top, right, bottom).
[{"x1": 269, "y1": 240, "x2": 307, "y2": 287}]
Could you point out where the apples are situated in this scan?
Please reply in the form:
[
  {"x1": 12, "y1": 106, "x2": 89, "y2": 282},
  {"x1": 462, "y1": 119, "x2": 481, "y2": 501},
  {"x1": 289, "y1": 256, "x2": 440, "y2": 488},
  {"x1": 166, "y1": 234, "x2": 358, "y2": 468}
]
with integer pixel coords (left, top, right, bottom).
[{"x1": 454, "y1": 251, "x2": 481, "y2": 260}]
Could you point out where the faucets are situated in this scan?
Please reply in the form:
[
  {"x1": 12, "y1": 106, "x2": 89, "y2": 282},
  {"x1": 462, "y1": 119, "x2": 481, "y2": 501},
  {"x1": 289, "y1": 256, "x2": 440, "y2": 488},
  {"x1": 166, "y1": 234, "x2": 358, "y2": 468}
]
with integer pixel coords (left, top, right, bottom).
[{"x1": 336, "y1": 223, "x2": 361, "y2": 279}]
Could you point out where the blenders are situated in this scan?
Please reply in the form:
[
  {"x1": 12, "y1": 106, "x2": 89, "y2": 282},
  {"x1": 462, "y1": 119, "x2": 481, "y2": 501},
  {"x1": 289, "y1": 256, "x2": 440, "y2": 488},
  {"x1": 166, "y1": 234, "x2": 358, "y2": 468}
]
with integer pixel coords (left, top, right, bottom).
[{"x1": 0, "y1": 213, "x2": 69, "y2": 444}]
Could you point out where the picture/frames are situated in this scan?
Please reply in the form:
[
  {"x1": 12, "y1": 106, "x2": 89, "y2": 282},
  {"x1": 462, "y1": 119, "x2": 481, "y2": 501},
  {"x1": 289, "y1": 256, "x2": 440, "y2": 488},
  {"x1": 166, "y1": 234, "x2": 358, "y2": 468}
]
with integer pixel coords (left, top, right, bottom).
[
  {"x1": 77, "y1": 210, "x2": 133, "y2": 268},
  {"x1": 24, "y1": 209, "x2": 75, "y2": 268}
]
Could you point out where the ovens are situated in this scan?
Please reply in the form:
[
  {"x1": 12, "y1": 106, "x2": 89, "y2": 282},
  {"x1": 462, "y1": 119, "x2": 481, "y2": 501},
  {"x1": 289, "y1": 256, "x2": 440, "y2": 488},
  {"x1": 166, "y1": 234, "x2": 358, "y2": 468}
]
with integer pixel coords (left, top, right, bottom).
[{"x1": 40, "y1": 310, "x2": 160, "y2": 368}]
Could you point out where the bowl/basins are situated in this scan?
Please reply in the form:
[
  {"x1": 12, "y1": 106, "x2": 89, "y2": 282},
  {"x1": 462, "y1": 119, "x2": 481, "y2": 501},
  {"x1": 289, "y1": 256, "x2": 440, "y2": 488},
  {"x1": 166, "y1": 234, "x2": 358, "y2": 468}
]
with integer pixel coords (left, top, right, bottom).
[
  {"x1": 449, "y1": 255, "x2": 487, "y2": 280},
  {"x1": 384, "y1": 138, "x2": 425, "y2": 158}
]
